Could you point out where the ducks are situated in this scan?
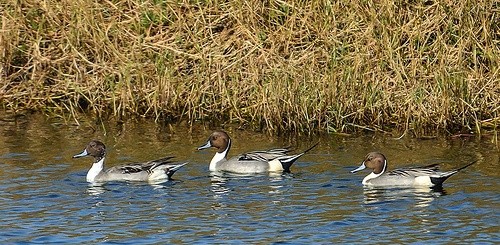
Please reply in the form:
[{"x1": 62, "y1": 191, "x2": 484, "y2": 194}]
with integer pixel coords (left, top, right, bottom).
[
  {"x1": 72, "y1": 139, "x2": 188, "y2": 186},
  {"x1": 351, "y1": 152, "x2": 478, "y2": 187},
  {"x1": 198, "y1": 131, "x2": 320, "y2": 173}
]
